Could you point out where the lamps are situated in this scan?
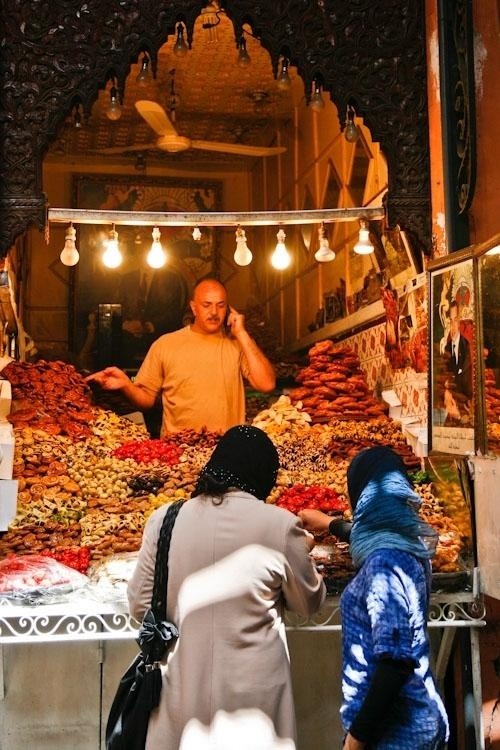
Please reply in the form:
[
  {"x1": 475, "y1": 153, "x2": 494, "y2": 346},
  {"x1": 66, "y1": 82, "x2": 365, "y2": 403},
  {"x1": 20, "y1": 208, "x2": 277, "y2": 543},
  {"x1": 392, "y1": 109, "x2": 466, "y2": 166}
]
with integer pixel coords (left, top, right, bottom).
[
  {"x1": 67, "y1": 16, "x2": 197, "y2": 128},
  {"x1": 58, "y1": 219, "x2": 81, "y2": 266},
  {"x1": 97, "y1": 221, "x2": 126, "y2": 269},
  {"x1": 199, "y1": 2, "x2": 219, "y2": 45},
  {"x1": 146, "y1": 225, "x2": 169, "y2": 270},
  {"x1": 352, "y1": 219, "x2": 374, "y2": 257},
  {"x1": 227, "y1": 10, "x2": 364, "y2": 144},
  {"x1": 312, "y1": 221, "x2": 337, "y2": 264},
  {"x1": 267, "y1": 225, "x2": 297, "y2": 272},
  {"x1": 230, "y1": 227, "x2": 253, "y2": 266}
]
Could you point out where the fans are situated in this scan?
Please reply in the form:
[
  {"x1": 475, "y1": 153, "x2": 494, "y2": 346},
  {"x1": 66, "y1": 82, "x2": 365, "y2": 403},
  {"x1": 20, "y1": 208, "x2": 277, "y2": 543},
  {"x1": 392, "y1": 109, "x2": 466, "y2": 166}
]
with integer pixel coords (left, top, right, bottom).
[{"x1": 88, "y1": 92, "x2": 286, "y2": 165}]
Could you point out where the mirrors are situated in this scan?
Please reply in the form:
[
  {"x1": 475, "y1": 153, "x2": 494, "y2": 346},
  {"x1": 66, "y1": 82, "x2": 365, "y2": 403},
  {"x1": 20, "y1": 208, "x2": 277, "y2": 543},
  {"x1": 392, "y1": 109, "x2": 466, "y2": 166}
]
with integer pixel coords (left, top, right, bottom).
[{"x1": 475, "y1": 234, "x2": 500, "y2": 448}]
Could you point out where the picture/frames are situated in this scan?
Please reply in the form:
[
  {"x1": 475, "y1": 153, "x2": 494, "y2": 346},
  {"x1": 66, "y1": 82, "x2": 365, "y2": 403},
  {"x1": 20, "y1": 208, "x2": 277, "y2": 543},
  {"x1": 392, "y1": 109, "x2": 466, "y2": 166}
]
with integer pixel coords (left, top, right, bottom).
[
  {"x1": 62, "y1": 168, "x2": 225, "y2": 382},
  {"x1": 426, "y1": 245, "x2": 482, "y2": 461},
  {"x1": 357, "y1": 182, "x2": 422, "y2": 290}
]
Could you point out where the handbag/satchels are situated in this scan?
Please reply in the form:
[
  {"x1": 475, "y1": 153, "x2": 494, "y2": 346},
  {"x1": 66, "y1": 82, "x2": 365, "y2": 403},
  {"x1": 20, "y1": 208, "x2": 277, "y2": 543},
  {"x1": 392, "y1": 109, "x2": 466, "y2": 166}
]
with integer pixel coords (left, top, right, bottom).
[{"x1": 105, "y1": 651, "x2": 161, "y2": 750}]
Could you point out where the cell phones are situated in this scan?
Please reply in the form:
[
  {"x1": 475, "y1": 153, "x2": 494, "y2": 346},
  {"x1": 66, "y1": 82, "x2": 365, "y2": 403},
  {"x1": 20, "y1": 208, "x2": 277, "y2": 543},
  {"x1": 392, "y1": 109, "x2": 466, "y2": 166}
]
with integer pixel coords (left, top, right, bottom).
[{"x1": 224, "y1": 304, "x2": 232, "y2": 338}]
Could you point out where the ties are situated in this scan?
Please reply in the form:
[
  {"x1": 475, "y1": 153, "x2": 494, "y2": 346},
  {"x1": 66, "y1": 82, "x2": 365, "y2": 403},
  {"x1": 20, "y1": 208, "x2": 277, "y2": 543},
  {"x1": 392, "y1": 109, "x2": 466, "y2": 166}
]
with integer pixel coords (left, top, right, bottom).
[{"x1": 452, "y1": 344, "x2": 456, "y2": 369}]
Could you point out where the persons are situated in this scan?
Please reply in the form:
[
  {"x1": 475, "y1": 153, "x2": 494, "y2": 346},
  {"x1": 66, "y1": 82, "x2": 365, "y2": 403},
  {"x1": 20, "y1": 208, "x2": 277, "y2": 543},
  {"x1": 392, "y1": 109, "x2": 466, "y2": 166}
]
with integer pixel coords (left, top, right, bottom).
[
  {"x1": 297, "y1": 447, "x2": 450, "y2": 750},
  {"x1": 84, "y1": 276, "x2": 276, "y2": 442},
  {"x1": 444, "y1": 302, "x2": 471, "y2": 395},
  {"x1": 127, "y1": 427, "x2": 327, "y2": 750}
]
